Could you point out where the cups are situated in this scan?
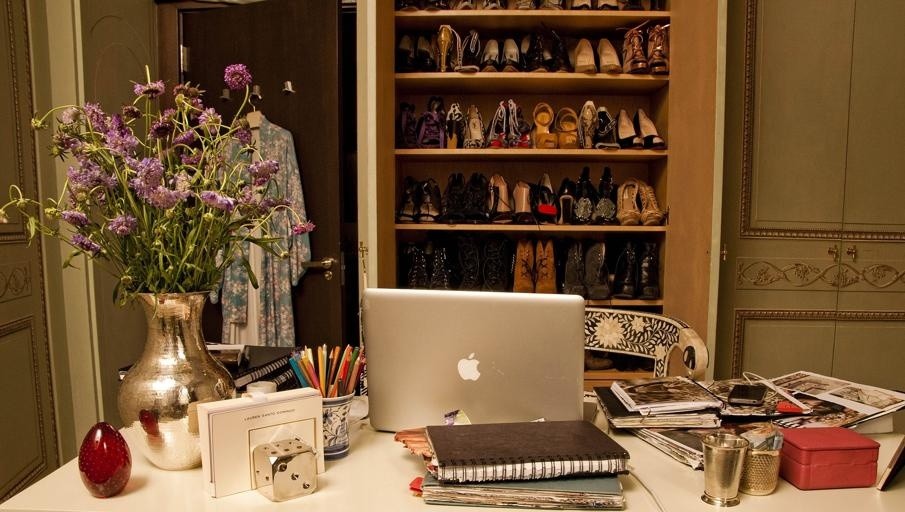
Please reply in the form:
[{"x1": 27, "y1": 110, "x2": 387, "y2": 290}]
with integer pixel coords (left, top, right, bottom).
[
  {"x1": 700, "y1": 433, "x2": 751, "y2": 506},
  {"x1": 323, "y1": 388, "x2": 356, "y2": 459}
]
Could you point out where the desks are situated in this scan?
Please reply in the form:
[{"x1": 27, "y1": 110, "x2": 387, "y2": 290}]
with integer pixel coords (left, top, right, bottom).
[{"x1": 0, "y1": 392, "x2": 905, "y2": 511}]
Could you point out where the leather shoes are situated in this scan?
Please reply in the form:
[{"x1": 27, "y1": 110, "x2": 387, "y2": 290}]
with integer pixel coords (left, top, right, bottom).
[{"x1": 394, "y1": 1, "x2": 669, "y2": 75}]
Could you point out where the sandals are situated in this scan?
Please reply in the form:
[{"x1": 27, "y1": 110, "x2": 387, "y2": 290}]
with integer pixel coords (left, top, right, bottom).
[
  {"x1": 395, "y1": 165, "x2": 665, "y2": 226},
  {"x1": 394, "y1": 95, "x2": 666, "y2": 150}
]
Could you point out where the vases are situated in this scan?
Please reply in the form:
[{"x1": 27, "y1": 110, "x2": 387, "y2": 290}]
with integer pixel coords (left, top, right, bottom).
[{"x1": 114, "y1": 287, "x2": 242, "y2": 475}]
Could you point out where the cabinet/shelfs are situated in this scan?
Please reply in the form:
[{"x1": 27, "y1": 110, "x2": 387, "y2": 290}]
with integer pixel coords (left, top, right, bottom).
[
  {"x1": 354, "y1": 0, "x2": 731, "y2": 394},
  {"x1": 710, "y1": 0, "x2": 904, "y2": 391}
]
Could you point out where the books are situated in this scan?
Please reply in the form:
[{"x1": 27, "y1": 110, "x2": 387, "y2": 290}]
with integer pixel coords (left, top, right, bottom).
[
  {"x1": 593, "y1": 371, "x2": 904, "y2": 471},
  {"x1": 420, "y1": 421, "x2": 631, "y2": 511},
  {"x1": 116, "y1": 342, "x2": 303, "y2": 398}
]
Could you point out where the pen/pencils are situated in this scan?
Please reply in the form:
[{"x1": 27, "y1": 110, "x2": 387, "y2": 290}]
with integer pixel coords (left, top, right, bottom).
[{"x1": 288, "y1": 343, "x2": 366, "y2": 397}]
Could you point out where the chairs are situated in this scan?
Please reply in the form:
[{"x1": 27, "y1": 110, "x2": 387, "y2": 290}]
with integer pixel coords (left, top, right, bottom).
[{"x1": 582, "y1": 305, "x2": 712, "y2": 398}]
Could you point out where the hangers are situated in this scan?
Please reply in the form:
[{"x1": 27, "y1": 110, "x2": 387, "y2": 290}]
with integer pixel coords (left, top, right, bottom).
[{"x1": 245, "y1": 88, "x2": 263, "y2": 113}]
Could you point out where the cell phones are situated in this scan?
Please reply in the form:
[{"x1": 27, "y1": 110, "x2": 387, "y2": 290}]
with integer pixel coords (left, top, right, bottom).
[{"x1": 728, "y1": 384, "x2": 768, "y2": 406}]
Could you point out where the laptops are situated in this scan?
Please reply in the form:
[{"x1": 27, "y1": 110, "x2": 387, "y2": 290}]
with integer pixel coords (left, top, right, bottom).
[{"x1": 361, "y1": 289, "x2": 597, "y2": 434}]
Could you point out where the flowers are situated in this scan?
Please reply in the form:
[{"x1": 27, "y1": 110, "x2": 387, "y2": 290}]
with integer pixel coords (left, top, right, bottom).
[{"x1": 0, "y1": 52, "x2": 320, "y2": 312}]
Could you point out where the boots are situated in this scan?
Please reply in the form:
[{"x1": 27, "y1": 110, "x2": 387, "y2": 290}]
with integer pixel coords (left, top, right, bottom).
[{"x1": 402, "y1": 236, "x2": 661, "y2": 301}]
